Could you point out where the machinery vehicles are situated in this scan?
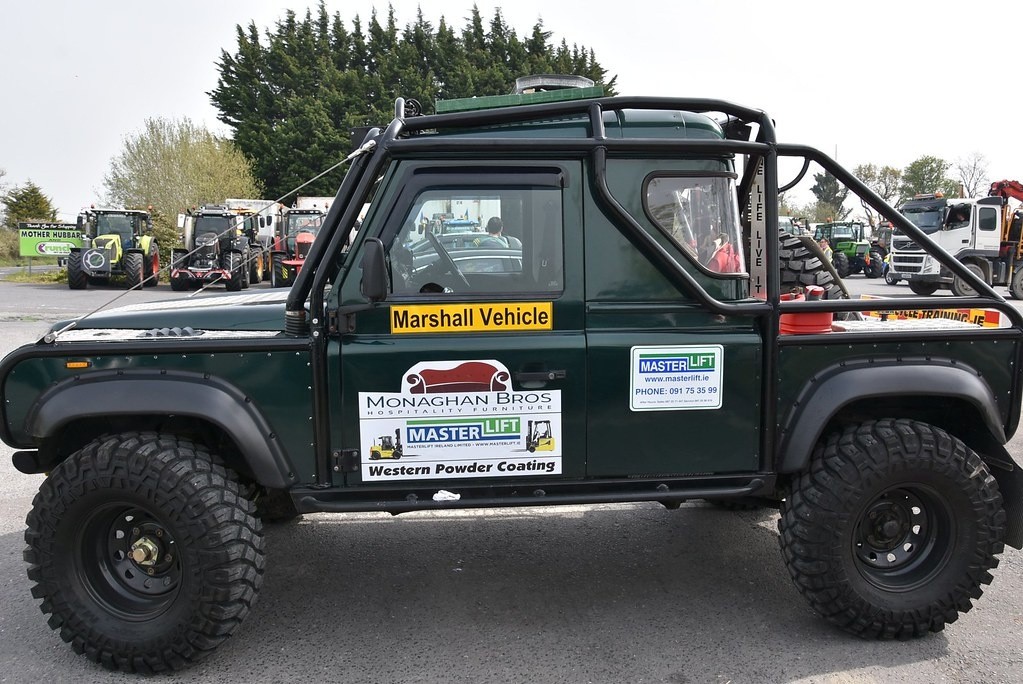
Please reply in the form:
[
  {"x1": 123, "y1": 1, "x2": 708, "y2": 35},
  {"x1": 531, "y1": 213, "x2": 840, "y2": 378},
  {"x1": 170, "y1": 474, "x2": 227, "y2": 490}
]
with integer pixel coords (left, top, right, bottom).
[
  {"x1": 812, "y1": 220, "x2": 883, "y2": 278},
  {"x1": 887, "y1": 179, "x2": 1023, "y2": 300},
  {"x1": 169, "y1": 202, "x2": 266, "y2": 291},
  {"x1": 67, "y1": 205, "x2": 161, "y2": 292},
  {"x1": 440, "y1": 218, "x2": 482, "y2": 235},
  {"x1": 268, "y1": 202, "x2": 329, "y2": 287}
]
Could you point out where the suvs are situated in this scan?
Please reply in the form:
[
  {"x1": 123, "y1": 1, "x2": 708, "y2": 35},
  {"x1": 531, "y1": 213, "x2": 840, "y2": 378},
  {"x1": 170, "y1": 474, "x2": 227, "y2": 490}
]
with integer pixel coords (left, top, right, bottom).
[{"x1": 0, "y1": 76, "x2": 1023, "y2": 680}]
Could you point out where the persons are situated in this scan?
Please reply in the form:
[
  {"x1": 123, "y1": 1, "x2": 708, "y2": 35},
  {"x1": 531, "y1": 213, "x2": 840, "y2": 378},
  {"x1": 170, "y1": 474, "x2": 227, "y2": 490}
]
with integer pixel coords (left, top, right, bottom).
[
  {"x1": 420, "y1": 217, "x2": 429, "y2": 239},
  {"x1": 440, "y1": 214, "x2": 446, "y2": 222},
  {"x1": 947, "y1": 212, "x2": 969, "y2": 230},
  {"x1": 468, "y1": 217, "x2": 509, "y2": 272},
  {"x1": 821, "y1": 238, "x2": 833, "y2": 263}
]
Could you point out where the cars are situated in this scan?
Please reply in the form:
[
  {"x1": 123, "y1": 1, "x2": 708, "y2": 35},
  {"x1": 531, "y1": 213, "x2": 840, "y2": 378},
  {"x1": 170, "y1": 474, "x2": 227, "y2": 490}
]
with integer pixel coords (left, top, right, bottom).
[
  {"x1": 404, "y1": 247, "x2": 524, "y2": 294},
  {"x1": 408, "y1": 231, "x2": 524, "y2": 274}
]
